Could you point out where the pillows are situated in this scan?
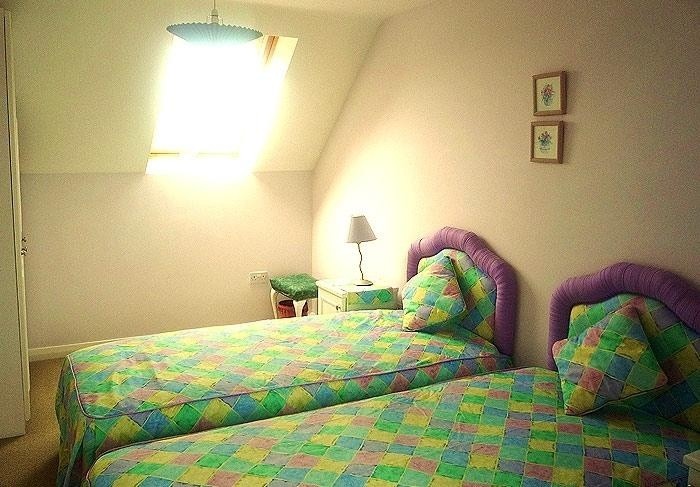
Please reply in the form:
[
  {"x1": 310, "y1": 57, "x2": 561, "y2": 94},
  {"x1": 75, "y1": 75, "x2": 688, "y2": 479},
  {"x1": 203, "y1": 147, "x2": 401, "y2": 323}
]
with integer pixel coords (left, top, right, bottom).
[
  {"x1": 401, "y1": 256, "x2": 463, "y2": 334},
  {"x1": 552, "y1": 303, "x2": 670, "y2": 419}
]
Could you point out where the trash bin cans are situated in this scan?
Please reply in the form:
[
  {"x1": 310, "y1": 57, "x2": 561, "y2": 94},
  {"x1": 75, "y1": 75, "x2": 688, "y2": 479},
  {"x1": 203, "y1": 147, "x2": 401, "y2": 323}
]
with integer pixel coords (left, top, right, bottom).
[{"x1": 277, "y1": 300, "x2": 308, "y2": 318}]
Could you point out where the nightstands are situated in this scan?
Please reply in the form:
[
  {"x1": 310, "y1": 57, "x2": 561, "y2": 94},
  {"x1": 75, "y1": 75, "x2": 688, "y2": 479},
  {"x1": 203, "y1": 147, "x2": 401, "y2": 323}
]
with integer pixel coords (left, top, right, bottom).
[
  {"x1": 680, "y1": 451, "x2": 700, "y2": 486},
  {"x1": 316, "y1": 278, "x2": 400, "y2": 315}
]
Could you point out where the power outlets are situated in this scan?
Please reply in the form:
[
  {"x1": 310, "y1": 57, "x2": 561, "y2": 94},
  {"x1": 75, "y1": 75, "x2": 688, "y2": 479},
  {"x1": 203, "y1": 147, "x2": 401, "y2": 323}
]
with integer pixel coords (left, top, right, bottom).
[{"x1": 248, "y1": 271, "x2": 269, "y2": 286}]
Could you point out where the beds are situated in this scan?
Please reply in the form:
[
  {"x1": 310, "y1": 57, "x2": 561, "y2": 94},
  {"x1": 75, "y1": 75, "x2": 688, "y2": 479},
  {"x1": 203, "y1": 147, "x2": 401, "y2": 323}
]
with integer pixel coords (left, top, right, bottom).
[
  {"x1": 55, "y1": 227, "x2": 521, "y2": 487},
  {"x1": 85, "y1": 263, "x2": 700, "y2": 487}
]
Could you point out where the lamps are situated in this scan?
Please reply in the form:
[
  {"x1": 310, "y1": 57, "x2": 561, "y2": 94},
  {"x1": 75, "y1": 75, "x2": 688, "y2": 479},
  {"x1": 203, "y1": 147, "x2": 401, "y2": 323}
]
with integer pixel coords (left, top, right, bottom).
[
  {"x1": 166, "y1": 0, "x2": 264, "y2": 53},
  {"x1": 345, "y1": 215, "x2": 378, "y2": 287}
]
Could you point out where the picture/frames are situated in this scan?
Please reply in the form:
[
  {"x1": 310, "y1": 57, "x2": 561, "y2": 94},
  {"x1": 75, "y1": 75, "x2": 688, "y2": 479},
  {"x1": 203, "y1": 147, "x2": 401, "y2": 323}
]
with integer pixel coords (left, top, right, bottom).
[{"x1": 530, "y1": 69, "x2": 567, "y2": 165}]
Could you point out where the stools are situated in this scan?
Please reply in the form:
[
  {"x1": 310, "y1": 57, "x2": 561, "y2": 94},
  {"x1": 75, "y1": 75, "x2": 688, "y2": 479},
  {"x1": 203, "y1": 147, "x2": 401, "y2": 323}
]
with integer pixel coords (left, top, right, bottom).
[{"x1": 270, "y1": 272, "x2": 319, "y2": 319}]
